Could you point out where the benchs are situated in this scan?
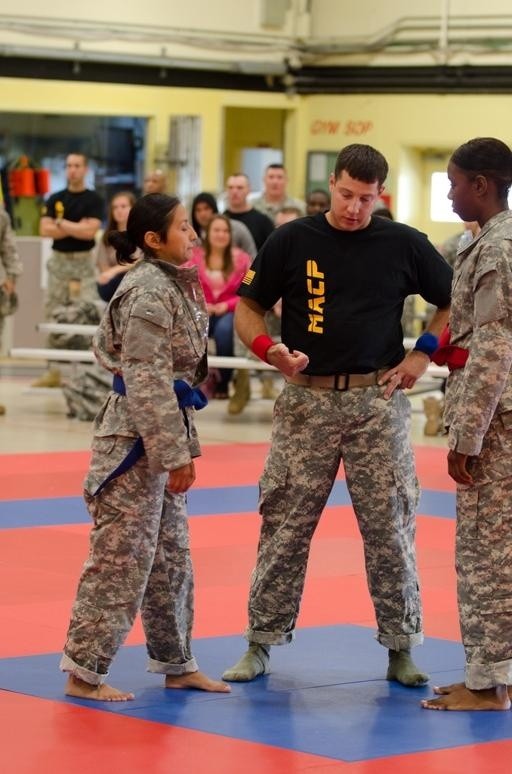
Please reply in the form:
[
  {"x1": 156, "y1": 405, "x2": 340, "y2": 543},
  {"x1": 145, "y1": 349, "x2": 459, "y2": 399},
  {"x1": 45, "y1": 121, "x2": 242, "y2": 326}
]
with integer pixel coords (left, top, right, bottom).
[
  {"x1": 7, "y1": 345, "x2": 289, "y2": 414},
  {"x1": 37, "y1": 322, "x2": 248, "y2": 356}
]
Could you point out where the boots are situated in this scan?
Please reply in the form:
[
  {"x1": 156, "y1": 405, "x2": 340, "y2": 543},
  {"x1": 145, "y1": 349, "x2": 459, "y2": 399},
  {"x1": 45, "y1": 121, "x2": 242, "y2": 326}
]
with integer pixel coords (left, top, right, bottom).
[
  {"x1": 423, "y1": 398, "x2": 441, "y2": 435},
  {"x1": 262, "y1": 376, "x2": 277, "y2": 398},
  {"x1": 229, "y1": 370, "x2": 250, "y2": 413},
  {"x1": 32, "y1": 369, "x2": 60, "y2": 387}
]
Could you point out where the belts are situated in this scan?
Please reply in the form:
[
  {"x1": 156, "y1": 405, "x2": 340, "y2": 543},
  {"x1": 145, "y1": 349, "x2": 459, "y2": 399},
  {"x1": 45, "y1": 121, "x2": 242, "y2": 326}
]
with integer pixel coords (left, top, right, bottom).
[{"x1": 284, "y1": 372, "x2": 381, "y2": 391}]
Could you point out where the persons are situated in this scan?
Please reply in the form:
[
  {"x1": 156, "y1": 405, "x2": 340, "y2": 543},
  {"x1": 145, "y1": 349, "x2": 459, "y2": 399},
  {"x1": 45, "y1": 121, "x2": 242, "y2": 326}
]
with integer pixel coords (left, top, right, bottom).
[
  {"x1": 58, "y1": 192, "x2": 231, "y2": 703},
  {"x1": 1, "y1": 153, "x2": 484, "y2": 435},
  {"x1": 220, "y1": 142, "x2": 457, "y2": 687},
  {"x1": 417, "y1": 135, "x2": 512, "y2": 714}
]
